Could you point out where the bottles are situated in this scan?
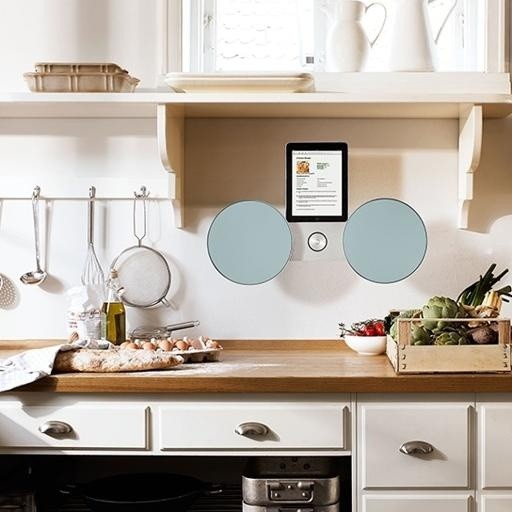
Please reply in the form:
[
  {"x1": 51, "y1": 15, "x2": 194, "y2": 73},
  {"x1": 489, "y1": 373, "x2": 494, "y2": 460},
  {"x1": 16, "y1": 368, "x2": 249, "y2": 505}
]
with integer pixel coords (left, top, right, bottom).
[{"x1": 101, "y1": 263, "x2": 126, "y2": 345}]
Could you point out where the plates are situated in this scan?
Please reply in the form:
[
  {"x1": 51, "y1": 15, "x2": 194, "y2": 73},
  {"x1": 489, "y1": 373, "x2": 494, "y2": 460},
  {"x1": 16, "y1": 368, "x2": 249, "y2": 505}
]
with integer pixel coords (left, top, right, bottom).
[{"x1": 162, "y1": 70, "x2": 312, "y2": 92}]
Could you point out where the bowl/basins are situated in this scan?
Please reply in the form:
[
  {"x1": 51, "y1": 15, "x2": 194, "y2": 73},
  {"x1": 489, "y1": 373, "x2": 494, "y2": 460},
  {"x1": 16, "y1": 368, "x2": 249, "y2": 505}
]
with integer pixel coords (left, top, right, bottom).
[{"x1": 343, "y1": 333, "x2": 388, "y2": 356}]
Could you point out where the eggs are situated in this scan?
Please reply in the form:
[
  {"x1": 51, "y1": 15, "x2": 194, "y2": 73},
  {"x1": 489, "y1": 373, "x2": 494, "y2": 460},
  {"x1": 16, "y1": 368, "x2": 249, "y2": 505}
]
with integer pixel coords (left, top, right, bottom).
[{"x1": 120, "y1": 335, "x2": 220, "y2": 351}]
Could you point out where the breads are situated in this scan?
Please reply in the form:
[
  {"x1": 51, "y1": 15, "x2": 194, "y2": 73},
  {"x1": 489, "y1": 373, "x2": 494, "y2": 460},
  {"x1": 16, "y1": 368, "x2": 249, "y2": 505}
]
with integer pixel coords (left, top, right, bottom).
[{"x1": 52, "y1": 348, "x2": 185, "y2": 371}]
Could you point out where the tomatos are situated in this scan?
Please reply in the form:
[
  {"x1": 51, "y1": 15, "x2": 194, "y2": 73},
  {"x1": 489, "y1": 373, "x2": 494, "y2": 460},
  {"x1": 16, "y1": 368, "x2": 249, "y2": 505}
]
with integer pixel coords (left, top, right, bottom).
[{"x1": 351, "y1": 321, "x2": 386, "y2": 336}]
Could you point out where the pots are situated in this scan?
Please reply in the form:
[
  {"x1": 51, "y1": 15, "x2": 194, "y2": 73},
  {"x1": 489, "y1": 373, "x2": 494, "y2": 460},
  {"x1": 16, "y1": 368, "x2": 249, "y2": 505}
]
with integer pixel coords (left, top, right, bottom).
[{"x1": 57, "y1": 472, "x2": 225, "y2": 511}]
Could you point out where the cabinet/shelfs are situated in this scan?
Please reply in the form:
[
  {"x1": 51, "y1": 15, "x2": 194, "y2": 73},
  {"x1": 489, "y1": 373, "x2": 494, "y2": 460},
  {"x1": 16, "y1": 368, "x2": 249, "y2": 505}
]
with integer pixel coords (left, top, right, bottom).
[
  {"x1": 355, "y1": 389, "x2": 511, "y2": 511},
  {"x1": 1, "y1": 390, "x2": 158, "y2": 458},
  {"x1": 158, "y1": 391, "x2": 355, "y2": 457}
]
